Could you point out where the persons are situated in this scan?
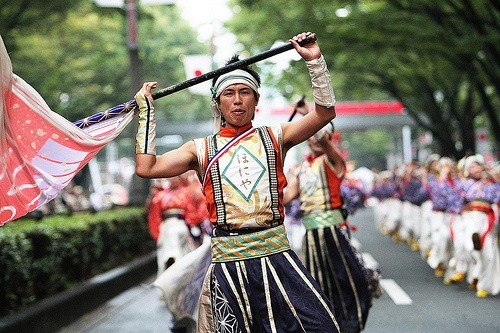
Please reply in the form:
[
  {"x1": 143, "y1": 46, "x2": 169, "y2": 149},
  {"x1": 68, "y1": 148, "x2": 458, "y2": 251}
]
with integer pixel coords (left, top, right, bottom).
[
  {"x1": 283, "y1": 99, "x2": 369, "y2": 333},
  {"x1": 284, "y1": 164, "x2": 382, "y2": 307},
  {"x1": 373, "y1": 155, "x2": 500, "y2": 297},
  {"x1": 133, "y1": 32, "x2": 341, "y2": 333},
  {"x1": 144, "y1": 170, "x2": 215, "y2": 333}
]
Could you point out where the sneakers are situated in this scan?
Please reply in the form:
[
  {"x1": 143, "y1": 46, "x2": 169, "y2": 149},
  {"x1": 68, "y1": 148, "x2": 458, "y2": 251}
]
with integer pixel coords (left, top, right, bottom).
[
  {"x1": 378, "y1": 226, "x2": 434, "y2": 256},
  {"x1": 166, "y1": 257, "x2": 175, "y2": 269},
  {"x1": 170, "y1": 315, "x2": 196, "y2": 333},
  {"x1": 370, "y1": 269, "x2": 382, "y2": 299},
  {"x1": 435, "y1": 263, "x2": 488, "y2": 298}
]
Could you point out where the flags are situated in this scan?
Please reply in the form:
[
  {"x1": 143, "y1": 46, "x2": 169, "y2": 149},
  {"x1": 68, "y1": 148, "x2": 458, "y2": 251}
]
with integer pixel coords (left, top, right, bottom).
[
  {"x1": 0, "y1": 34, "x2": 139, "y2": 227},
  {"x1": 183, "y1": 53, "x2": 213, "y2": 96}
]
